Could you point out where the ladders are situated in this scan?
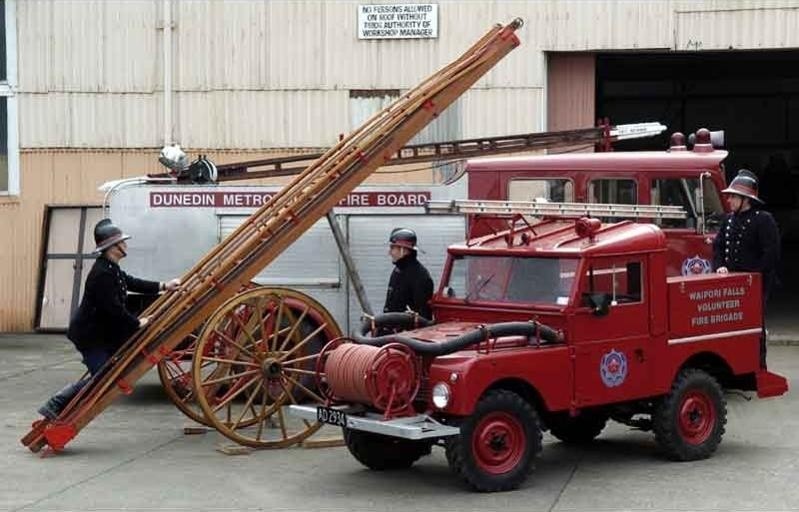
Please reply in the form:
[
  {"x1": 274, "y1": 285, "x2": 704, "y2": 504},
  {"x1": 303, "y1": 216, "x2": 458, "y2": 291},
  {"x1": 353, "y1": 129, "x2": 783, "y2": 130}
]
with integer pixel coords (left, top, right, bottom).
[{"x1": 422, "y1": 201, "x2": 689, "y2": 218}]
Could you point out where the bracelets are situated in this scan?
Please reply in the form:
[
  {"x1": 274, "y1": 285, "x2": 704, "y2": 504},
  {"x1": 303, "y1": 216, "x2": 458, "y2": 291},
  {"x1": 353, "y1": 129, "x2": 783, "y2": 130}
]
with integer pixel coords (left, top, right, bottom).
[{"x1": 163, "y1": 282, "x2": 165, "y2": 290}]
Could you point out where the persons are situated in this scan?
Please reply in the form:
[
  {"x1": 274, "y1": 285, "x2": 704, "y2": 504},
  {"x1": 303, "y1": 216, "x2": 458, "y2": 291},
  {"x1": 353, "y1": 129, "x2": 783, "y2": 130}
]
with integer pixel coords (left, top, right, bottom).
[
  {"x1": 38, "y1": 218, "x2": 181, "y2": 420},
  {"x1": 714, "y1": 169, "x2": 780, "y2": 371},
  {"x1": 371, "y1": 228, "x2": 433, "y2": 338}
]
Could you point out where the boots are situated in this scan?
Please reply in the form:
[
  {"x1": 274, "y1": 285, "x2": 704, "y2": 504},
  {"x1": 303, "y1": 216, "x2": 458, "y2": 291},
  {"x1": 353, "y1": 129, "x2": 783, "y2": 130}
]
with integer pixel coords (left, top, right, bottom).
[{"x1": 38, "y1": 397, "x2": 64, "y2": 421}]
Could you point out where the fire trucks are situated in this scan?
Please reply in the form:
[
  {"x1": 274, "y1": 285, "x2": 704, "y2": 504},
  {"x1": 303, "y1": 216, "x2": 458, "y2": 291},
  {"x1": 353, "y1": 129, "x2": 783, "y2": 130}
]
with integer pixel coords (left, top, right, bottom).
[{"x1": 98, "y1": 117, "x2": 732, "y2": 404}]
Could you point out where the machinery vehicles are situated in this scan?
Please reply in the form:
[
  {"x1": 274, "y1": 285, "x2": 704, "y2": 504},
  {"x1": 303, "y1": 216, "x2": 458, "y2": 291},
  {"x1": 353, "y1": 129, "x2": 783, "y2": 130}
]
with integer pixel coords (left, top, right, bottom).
[{"x1": 288, "y1": 213, "x2": 789, "y2": 493}]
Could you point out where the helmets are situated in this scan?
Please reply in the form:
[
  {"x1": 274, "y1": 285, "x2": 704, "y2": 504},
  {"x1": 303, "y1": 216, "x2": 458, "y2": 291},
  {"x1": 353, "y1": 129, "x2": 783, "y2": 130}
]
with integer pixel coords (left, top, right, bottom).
[
  {"x1": 720, "y1": 168, "x2": 766, "y2": 206},
  {"x1": 389, "y1": 228, "x2": 424, "y2": 252},
  {"x1": 89, "y1": 218, "x2": 130, "y2": 255}
]
